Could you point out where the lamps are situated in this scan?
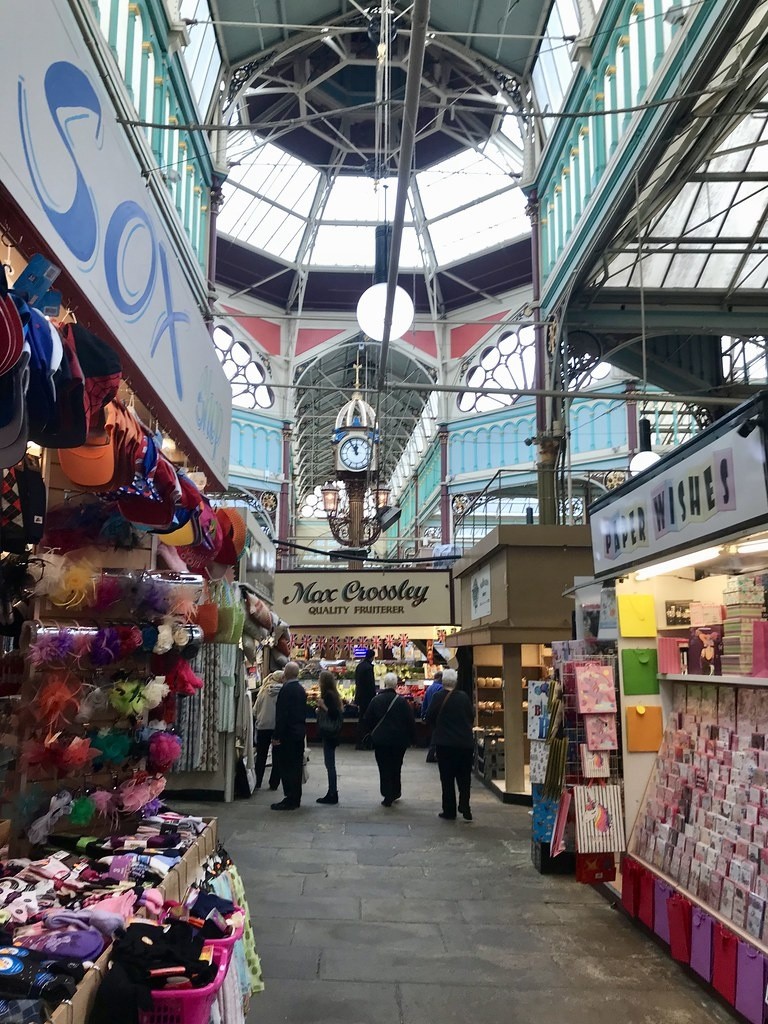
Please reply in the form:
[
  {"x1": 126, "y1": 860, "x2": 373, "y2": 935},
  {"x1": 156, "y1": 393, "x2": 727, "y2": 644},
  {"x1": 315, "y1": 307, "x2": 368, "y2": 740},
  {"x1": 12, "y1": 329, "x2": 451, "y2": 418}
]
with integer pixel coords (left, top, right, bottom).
[
  {"x1": 357, "y1": 185, "x2": 415, "y2": 340},
  {"x1": 629, "y1": 416, "x2": 660, "y2": 475},
  {"x1": 634, "y1": 542, "x2": 725, "y2": 581},
  {"x1": 726, "y1": 538, "x2": 768, "y2": 555}
]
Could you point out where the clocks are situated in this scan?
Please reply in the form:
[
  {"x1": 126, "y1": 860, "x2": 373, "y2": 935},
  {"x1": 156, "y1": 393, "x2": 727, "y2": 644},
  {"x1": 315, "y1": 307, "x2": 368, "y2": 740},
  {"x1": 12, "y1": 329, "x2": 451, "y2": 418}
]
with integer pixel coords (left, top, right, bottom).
[{"x1": 337, "y1": 437, "x2": 375, "y2": 473}]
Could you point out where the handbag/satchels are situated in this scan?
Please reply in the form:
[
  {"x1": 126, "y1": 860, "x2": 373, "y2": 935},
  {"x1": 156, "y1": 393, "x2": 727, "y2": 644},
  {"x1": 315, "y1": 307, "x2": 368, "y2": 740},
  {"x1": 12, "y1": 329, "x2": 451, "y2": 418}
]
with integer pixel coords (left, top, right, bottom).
[
  {"x1": 316, "y1": 711, "x2": 339, "y2": 739},
  {"x1": 526, "y1": 664, "x2": 627, "y2": 859},
  {"x1": 356, "y1": 720, "x2": 373, "y2": 750}
]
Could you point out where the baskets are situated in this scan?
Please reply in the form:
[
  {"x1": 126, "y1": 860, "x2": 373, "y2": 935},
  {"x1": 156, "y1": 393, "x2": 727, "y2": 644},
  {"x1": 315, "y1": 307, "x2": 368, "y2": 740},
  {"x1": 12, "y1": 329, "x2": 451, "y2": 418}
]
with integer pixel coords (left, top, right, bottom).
[{"x1": 136, "y1": 902, "x2": 246, "y2": 1024}]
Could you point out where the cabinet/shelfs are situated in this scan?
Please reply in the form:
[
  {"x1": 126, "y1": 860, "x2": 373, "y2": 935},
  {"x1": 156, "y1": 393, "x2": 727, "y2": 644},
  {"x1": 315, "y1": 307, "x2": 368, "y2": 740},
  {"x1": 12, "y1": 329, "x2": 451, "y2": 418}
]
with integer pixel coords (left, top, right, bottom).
[
  {"x1": 475, "y1": 677, "x2": 528, "y2": 733},
  {"x1": 657, "y1": 623, "x2": 768, "y2": 686},
  {"x1": 627, "y1": 717, "x2": 768, "y2": 958}
]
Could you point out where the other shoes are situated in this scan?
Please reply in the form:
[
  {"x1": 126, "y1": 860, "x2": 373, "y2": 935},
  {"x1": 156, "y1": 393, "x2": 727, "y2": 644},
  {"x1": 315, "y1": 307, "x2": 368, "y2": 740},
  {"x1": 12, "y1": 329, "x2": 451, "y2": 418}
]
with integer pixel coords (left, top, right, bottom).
[
  {"x1": 271, "y1": 798, "x2": 294, "y2": 810},
  {"x1": 426, "y1": 757, "x2": 438, "y2": 763},
  {"x1": 382, "y1": 790, "x2": 401, "y2": 807},
  {"x1": 458, "y1": 807, "x2": 472, "y2": 820},
  {"x1": 270, "y1": 784, "x2": 277, "y2": 790},
  {"x1": 439, "y1": 812, "x2": 455, "y2": 820},
  {"x1": 256, "y1": 777, "x2": 262, "y2": 788},
  {"x1": 294, "y1": 799, "x2": 300, "y2": 807},
  {"x1": 317, "y1": 790, "x2": 338, "y2": 804}
]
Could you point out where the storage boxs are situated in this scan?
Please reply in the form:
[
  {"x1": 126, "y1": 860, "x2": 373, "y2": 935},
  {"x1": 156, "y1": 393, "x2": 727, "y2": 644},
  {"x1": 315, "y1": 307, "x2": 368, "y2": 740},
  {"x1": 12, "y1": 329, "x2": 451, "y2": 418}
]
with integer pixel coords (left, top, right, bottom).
[
  {"x1": 138, "y1": 945, "x2": 227, "y2": 1024},
  {"x1": 204, "y1": 905, "x2": 245, "y2": 972},
  {"x1": 658, "y1": 573, "x2": 768, "y2": 678},
  {"x1": 530, "y1": 837, "x2": 575, "y2": 876}
]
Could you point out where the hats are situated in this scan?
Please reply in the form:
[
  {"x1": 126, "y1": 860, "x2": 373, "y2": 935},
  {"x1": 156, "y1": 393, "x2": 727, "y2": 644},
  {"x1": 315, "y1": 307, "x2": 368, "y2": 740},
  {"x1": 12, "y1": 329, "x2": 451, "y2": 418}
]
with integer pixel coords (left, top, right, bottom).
[{"x1": 0, "y1": 263, "x2": 248, "y2": 580}]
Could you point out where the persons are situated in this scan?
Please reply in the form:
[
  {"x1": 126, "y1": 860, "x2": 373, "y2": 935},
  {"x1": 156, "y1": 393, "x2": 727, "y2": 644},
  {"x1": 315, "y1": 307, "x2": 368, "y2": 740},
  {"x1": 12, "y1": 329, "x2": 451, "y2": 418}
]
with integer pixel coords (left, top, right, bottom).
[
  {"x1": 252, "y1": 670, "x2": 288, "y2": 791},
  {"x1": 353, "y1": 650, "x2": 376, "y2": 752},
  {"x1": 271, "y1": 660, "x2": 307, "y2": 811},
  {"x1": 421, "y1": 672, "x2": 444, "y2": 763},
  {"x1": 425, "y1": 668, "x2": 476, "y2": 820},
  {"x1": 364, "y1": 672, "x2": 416, "y2": 808},
  {"x1": 316, "y1": 673, "x2": 343, "y2": 805}
]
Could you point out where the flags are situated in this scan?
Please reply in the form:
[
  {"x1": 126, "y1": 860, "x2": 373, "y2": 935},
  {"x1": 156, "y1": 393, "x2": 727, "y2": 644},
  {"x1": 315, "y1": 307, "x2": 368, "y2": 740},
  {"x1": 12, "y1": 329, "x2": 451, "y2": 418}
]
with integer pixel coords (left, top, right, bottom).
[
  {"x1": 287, "y1": 634, "x2": 298, "y2": 649},
  {"x1": 329, "y1": 636, "x2": 341, "y2": 650},
  {"x1": 357, "y1": 636, "x2": 369, "y2": 649},
  {"x1": 299, "y1": 634, "x2": 312, "y2": 649},
  {"x1": 438, "y1": 630, "x2": 446, "y2": 644},
  {"x1": 399, "y1": 634, "x2": 409, "y2": 648},
  {"x1": 344, "y1": 636, "x2": 354, "y2": 650},
  {"x1": 385, "y1": 635, "x2": 395, "y2": 650},
  {"x1": 315, "y1": 636, "x2": 327, "y2": 651},
  {"x1": 371, "y1": 636, "x2": 382, "y2": 649}
]
